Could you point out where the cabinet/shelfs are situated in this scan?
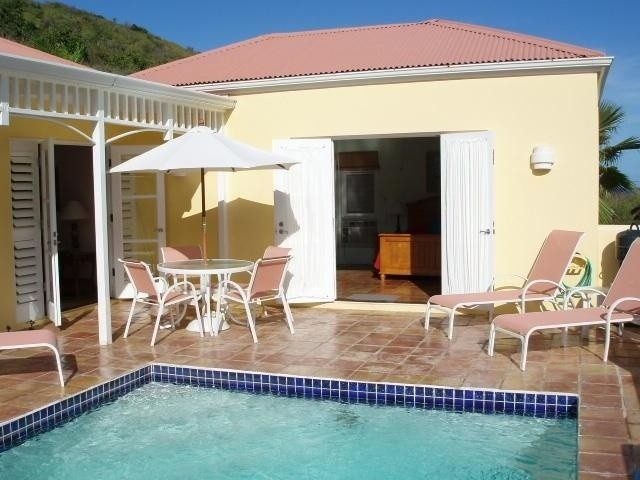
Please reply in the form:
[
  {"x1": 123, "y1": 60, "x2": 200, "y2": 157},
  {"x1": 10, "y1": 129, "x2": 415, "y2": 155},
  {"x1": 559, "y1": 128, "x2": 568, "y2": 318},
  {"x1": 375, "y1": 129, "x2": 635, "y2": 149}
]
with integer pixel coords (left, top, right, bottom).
[{"x1": 376, "y1": 231, "x2": 441, "y2": 280}]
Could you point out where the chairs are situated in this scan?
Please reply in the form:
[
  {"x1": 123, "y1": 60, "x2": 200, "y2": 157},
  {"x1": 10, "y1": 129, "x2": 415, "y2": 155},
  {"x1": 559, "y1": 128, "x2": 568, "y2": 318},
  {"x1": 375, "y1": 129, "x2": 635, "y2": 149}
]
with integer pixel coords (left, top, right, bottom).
[
  {"x1": 116, "y1": 244, "x2": 294, "y2": 346},
  {"x1": 0, "y1": 329, "x2": 67, "y2": 388},
  {"x1": 423, "y1": 230, "x2": 639, "y2": 371}
]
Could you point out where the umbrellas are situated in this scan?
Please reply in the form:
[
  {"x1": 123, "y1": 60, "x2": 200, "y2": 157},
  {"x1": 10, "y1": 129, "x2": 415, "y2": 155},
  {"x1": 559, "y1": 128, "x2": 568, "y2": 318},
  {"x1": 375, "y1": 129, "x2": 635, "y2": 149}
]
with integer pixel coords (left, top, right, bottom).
[{"x1": 109, "y1": 123, "x2": 300, "y2": 264}]
